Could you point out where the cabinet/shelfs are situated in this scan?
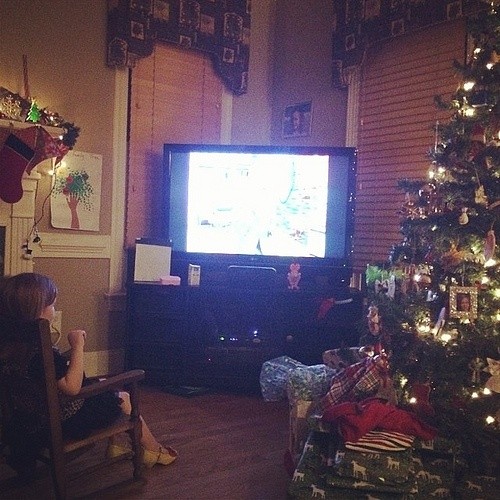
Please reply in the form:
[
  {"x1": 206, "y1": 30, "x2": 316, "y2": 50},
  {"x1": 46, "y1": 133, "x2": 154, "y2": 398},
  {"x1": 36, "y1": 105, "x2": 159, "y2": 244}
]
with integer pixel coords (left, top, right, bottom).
[{"x1": 126, "y1": 281, "x2": 364, "y2": 397}]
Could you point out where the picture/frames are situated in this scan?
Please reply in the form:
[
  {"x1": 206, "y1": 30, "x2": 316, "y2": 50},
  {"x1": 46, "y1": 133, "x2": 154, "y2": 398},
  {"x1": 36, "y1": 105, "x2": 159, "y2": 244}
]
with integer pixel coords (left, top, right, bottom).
[
  {"x1": 449, "y1": 286, "x2": 478, "y2": 319},
  {"x1": 281, "y1": 100, "x2": 314, "y2": 139}
]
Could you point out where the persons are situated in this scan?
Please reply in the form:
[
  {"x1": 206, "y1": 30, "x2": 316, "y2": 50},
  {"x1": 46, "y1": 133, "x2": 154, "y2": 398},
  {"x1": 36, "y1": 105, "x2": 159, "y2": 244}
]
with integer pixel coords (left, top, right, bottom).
[
  {"x1": 290, "y1": 110, "x2": 304, "y2": 135},
  {"x1": 459, "y1": 294, "x2": 471, "y2": 312},
  {"x1": 1, "y1": 272, "x2": 179, "y2": 470}
]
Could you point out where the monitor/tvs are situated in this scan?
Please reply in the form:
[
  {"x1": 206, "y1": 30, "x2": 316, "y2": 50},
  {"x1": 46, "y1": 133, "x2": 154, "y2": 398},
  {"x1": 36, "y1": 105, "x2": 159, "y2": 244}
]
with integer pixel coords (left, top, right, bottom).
[{"x1": 160, "y1": 142, "x2": 357, "y2": 286}]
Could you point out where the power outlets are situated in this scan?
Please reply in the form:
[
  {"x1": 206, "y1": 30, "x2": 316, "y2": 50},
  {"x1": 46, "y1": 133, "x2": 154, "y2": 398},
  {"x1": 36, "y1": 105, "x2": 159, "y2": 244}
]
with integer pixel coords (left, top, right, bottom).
[{"x1": 50, "y1": 311, "x2": 62, "y2": 333}]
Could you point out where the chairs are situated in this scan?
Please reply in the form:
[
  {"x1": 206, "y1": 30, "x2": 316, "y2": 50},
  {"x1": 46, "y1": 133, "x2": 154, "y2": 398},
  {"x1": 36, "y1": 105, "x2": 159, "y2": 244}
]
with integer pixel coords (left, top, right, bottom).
[{"x1": 0, "y1": 319, "x2": 148, "y2": 500}]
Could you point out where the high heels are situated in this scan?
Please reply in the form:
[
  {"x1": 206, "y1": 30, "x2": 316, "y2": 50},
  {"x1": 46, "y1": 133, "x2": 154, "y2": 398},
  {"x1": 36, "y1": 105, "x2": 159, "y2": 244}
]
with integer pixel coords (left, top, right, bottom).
[
  {"x1": 104, "y1": 442, "x2": 132, "y2": 461},
  {"x1": 136, "y1": 442, "x2": 179, "y2": 469}
]
